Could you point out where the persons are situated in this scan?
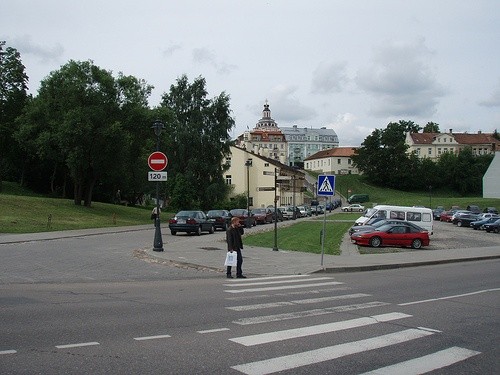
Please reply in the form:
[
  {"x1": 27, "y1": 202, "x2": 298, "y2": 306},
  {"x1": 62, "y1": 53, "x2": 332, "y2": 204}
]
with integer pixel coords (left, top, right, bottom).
[
  {"x1": 226, "y1": 217, "x2": 247, "y2": 278},
  {"x1": 152, "y1": 205, "x2": 161, "y2": 227}
]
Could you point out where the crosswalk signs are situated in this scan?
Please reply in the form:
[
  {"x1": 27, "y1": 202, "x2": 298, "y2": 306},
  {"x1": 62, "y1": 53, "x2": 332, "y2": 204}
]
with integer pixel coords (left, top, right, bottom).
[{"x1": 317, "y1": 175, "x2": 335, "y2": 196}]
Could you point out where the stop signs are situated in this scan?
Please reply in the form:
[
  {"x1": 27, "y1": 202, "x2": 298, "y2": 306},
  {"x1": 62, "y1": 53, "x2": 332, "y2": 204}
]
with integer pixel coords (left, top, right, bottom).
[{"x1": 148, "y1": 152, "x2": 168, "y2": 171}]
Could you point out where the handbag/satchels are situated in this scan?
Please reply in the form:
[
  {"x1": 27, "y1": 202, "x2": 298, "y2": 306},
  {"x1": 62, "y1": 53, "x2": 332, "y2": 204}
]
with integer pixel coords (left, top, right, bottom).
[
  {"x1": 151, "y1": 214, "x2": 154, "y2": 220},
  {"x1": 224, "y1": 251, "x2": 237, "y2": 266}
]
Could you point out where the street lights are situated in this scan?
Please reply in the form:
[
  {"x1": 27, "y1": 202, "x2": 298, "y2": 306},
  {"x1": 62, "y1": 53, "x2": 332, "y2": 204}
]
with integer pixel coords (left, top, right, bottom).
[
  {"x1": 245, "y1": 161, "x2": 252, "y2": 228},
  {"x1": 151, "y1": 114, "x2": 164, "y2": 252}
]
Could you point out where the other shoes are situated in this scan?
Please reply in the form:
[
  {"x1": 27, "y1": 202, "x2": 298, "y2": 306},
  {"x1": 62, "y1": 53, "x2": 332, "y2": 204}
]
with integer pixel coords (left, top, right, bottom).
[
  {"x1": 227, "y1": 274, "x2": 233, "y2": 278},
  {"x1": 237, "y1": 274, "x2": 246, "y2": 278}
]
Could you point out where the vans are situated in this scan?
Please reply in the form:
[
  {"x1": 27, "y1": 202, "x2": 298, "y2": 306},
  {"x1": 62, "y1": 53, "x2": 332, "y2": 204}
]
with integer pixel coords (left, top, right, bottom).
[
  {"x1": 354, "y1": 205, "x2": 434, "y2": 235},
  {"x1": 348, "y1": 194, "x2": 369, "y2": 204}
]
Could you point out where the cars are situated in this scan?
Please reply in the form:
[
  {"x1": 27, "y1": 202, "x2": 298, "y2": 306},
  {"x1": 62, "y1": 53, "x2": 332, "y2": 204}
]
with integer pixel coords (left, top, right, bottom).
[
  {"x1": 267, "y1": 207, "x2": 283, "y2": 222},
  {"x1": 432, "y1": 205, "x2": 500, "y2": 233},
  {"x1": 168, "y1": 210, "x2": 216, "y2": 236},
  {"x1": 251, "y1": 208, "x2": 273, "y2": 224},
  {"x1": 364, "y1": 208, "x2": 382, "y2": 218},
  {"x1": 230, "y1": 209, "x2": 257, "y2": 226},
  {"x1": 206, "y1": 210, "x2": 232, "y2": 230},
  {"x1": 350, "y1": 224, "x2": 430, "y2": 249},
  {"x1": 310, "y1": 199, "x2": 342, "y2": 215},
  {"x1": 341, "y1": 204, "x2": 364, "y2": 212},
  {"x1": 348, "y1": 219, "x2": 428, "y2": 236},
  {"x1": 278, "y1": 205, "x2": 312, "y2": 220}
]
